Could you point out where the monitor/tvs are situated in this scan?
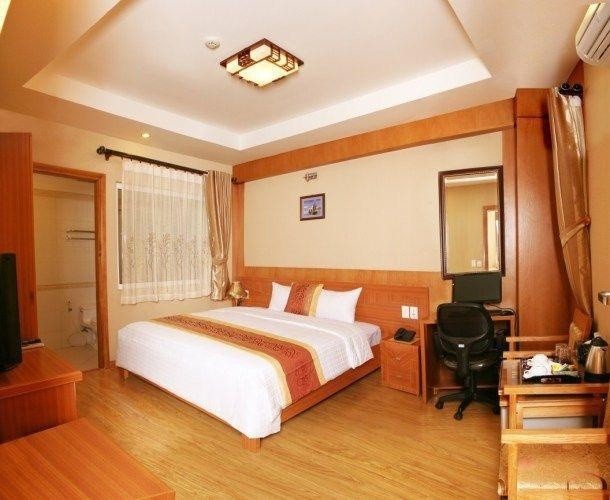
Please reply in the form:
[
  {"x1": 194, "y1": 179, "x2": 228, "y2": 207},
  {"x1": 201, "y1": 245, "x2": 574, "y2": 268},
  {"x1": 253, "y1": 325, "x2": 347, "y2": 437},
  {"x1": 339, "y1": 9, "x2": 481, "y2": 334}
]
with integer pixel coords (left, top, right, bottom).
[
  {"x1": 0, "y1": 253, "x2": 23, "y2": 376},
  {"x1": 452, "y1": 271, "x2": 502, "y2": 303}
]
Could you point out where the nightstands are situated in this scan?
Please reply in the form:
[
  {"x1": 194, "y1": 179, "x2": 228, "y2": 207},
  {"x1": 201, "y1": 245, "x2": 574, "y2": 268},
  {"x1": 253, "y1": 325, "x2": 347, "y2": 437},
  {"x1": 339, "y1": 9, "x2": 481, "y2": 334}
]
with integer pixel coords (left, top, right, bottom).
[{"x1": 380, "y1": 335, "x2": 421, "y2": 399}]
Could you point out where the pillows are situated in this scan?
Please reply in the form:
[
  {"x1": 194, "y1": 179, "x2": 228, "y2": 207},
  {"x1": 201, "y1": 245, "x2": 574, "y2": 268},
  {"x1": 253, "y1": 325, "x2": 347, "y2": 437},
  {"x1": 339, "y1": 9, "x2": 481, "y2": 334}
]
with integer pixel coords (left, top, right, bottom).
[
  {"x1": 269, "y1": 280, "x2": 362, "y2": 326},
  {"x1": 569, "y1": 321, "x2": 582, "y2": 348}
]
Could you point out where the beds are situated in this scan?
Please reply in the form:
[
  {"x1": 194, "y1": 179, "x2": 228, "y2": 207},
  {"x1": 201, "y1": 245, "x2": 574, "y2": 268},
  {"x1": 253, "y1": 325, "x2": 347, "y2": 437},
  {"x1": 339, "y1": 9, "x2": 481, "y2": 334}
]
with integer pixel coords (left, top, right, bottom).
[{"x1": 116, "y1": 275, "x2": 429, "y2": 452}]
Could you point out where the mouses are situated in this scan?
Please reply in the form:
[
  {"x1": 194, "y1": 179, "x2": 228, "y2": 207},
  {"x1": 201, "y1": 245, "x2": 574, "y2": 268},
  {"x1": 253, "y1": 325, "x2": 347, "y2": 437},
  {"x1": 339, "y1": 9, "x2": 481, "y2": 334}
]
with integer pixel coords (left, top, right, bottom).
[{"x1": 501, "y1": 311, "x2": 514, "y2": 315}]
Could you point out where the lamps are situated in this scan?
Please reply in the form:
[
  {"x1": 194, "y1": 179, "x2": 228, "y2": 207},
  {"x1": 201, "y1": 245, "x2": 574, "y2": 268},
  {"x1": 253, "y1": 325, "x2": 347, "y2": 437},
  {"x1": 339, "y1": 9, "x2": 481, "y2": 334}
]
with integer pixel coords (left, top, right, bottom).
[
  {"x1": 221, "y1": 39, "x2": 305, "y2": 87},
  {"x1": 228, "y1": 281, "x2": 247, "y2": 306}
]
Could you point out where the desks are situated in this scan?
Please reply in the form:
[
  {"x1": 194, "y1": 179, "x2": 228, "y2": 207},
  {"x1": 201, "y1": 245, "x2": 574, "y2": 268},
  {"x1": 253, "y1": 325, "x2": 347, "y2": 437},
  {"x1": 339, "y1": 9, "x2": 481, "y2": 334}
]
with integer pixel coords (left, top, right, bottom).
[
  {"x1": 420, "y1": 313, "x2": 516, "y2": 405},
  {"x1": 0, "y1": 418, "x2": 175, "y2": 500},
  {"x1": 0, "y1": 342, "x2": 83, "y2": 443}
]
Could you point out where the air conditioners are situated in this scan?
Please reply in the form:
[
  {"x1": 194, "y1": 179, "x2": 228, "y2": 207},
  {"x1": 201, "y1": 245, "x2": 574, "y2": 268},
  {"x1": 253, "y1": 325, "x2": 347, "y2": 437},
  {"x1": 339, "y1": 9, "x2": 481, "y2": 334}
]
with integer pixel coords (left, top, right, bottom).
[{"x1": 575, "y1": 3, "x2": 610, "y2": 66}]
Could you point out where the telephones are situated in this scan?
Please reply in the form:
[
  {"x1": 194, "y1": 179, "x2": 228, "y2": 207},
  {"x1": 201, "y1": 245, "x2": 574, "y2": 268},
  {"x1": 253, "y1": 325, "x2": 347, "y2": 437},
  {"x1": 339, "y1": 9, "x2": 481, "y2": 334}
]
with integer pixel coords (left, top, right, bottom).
[{"x1": 394, "y1": 327, "x2": 416, "y2": 342}]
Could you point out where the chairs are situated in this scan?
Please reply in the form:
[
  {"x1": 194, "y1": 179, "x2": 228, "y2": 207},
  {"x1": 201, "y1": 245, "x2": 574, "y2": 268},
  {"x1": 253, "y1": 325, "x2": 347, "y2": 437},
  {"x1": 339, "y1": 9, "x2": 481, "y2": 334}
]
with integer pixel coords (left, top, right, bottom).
[
  {"x1": 435, "y1": 303, "x2": 509, "y2": 420},
  {"x1": 497, "y1": 307, "x2": 610, "y2": 500}
]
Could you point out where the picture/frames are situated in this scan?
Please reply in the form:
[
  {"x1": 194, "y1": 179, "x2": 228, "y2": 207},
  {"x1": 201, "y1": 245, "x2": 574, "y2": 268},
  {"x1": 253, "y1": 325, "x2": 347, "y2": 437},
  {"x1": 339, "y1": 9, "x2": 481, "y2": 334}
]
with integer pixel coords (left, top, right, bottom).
[{"x1": 299, "y1": 193, "x2": 325, "y2": 221}]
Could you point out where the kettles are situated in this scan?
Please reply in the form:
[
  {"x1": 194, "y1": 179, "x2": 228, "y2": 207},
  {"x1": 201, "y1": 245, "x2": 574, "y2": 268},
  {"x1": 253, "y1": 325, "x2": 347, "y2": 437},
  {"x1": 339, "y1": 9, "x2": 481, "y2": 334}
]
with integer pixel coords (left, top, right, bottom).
[{"x1": 584, "y1": 336, "x2": 609, "y2": 382}]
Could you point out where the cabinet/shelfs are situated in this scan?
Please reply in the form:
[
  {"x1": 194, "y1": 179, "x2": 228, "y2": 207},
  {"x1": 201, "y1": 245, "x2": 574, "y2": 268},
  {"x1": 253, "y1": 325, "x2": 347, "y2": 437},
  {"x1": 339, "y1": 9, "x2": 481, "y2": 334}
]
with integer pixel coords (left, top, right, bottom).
[{"x1": 0, "y1": 132, "x2": 38, "y2": 341}]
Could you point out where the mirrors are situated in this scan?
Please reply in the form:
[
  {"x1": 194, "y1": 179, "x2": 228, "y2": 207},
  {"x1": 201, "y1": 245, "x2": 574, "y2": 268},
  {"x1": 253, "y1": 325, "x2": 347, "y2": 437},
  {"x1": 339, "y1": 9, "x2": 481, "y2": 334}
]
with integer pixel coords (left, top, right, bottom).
[{"x1": 438, "y1": 166, "x2": 505, "y2": 280}]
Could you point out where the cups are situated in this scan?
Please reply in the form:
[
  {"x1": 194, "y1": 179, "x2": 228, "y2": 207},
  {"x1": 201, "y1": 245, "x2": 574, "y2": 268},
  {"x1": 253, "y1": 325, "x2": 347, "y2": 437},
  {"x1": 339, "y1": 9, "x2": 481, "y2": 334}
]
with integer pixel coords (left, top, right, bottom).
[
  {"x1": 523, "y1": 354, "x2": 548, "y2": 378},
  {"x1": 555, "y1": 343, "x2": 578, "y2": 370}
]
